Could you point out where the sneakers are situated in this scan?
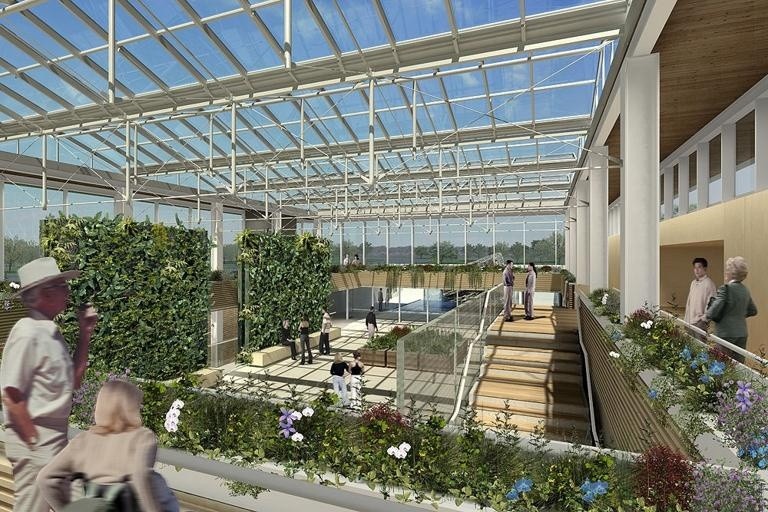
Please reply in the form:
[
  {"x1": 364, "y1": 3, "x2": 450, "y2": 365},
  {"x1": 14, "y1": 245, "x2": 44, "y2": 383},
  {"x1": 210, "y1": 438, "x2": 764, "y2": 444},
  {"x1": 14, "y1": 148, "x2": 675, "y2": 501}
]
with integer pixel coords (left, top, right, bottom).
[
  {"x1": 292, "y1": 350, "x2": 331, "y2": 365},
  {"x1": 504, "y1": 315, "x2": 535, "y2": 322}
]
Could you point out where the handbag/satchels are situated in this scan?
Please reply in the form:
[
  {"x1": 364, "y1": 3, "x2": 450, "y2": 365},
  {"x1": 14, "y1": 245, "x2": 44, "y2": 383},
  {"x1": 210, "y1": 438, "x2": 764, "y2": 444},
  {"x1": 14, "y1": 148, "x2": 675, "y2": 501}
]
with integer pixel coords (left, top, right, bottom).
[{"x1": 705, "y1": 296, "x2": 728, "y2": 324}]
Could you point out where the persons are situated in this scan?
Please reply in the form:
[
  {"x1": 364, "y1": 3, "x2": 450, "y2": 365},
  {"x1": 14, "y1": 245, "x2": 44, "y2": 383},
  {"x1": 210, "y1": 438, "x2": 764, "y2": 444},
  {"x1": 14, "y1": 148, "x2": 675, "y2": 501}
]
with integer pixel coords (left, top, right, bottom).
[
  {"x1": 330, "y1": 353, "x2": 351, "y2": 408},
  {"x1": 683, "y1": 257, "x2": 718, "y2": 344},
  {"x1": 352, "y1": 254, "x2": 360, "y2": 266},
  {"x1": 36, "y1": 379, "x2": 182, "y2": 512},
  {"x1": 503, "y1": 260, "x2": 516, "y2": 323},
  {"x1": 700, "y1": 256, "x2": 758, "y2": 365},
  {"x1": 318, "y1": 304, "x2": 332, "y2": 356},
  {"x1": 297, "y1": 315, "x2": 313, "y2": 365},
  {"x1": 378, "y1": 288, "x2": 382, "y2": 312},
  {"x1": 283, "y1": 319, "x2": 297, "y2": 359},
  {"x1": 365, "y1": 306, "x2": 378, "y2": 340},
  {"x1": 343, "y1": 254, "x2": 350, "y2": 266},
  {"x1": 0, "y1": 256, "x2": 99, "y2": 512},
  {"x1": 523, "y1": 263, "x2": 538, "y2": 322},
  {"x1": 348, "y1": 351, "x2": 365, "y2": 410},
  {"x1": 385, "y1": 289, "x2": 390, "y2": 311}
]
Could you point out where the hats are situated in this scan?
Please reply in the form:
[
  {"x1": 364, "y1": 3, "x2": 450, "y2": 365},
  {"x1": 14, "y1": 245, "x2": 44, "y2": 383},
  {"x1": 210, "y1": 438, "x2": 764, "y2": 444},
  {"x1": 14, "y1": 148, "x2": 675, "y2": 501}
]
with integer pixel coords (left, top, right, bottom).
[{"x1": 8, "y1": 256, "x2": 81, "y2": 301}]
